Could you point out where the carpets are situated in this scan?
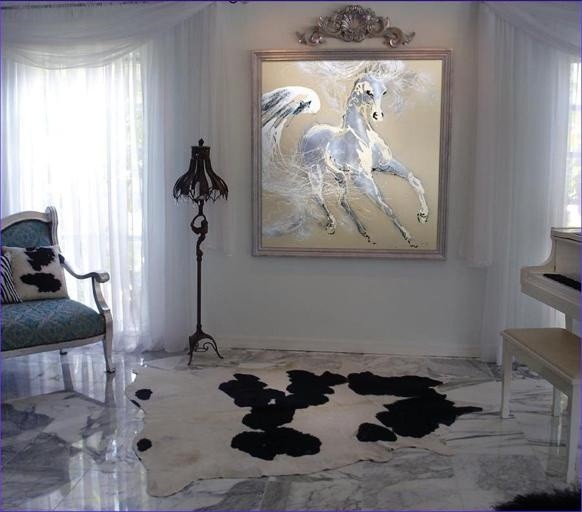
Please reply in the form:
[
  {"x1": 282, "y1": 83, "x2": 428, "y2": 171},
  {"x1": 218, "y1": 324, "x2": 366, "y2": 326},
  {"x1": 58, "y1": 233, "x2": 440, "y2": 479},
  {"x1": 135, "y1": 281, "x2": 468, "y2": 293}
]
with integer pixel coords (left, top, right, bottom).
[{"x1": 129, "y1": 352, "x2": 501, "y2": 500}]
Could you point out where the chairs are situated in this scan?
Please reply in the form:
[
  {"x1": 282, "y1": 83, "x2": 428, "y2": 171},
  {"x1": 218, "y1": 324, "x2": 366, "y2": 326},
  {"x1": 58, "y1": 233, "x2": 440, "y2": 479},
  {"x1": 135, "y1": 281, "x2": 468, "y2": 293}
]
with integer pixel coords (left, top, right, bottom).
[{"x1": 0, "y1": 206, "x2": 117, "y2": 375}]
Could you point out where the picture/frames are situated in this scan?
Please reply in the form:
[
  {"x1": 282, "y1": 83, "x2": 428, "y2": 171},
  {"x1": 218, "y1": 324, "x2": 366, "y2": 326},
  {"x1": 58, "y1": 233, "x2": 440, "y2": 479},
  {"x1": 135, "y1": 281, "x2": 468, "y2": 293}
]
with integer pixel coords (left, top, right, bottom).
[{"x1": 249, "y1": 48, "x2": 456, "y2": 263}]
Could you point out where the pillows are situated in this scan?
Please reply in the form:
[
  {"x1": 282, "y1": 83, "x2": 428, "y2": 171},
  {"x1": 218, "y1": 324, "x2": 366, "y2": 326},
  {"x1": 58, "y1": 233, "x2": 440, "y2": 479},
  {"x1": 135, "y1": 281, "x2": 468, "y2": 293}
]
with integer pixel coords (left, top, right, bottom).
[{"x1": 0, "y1": 243, "x2": 69, "y2": 305}]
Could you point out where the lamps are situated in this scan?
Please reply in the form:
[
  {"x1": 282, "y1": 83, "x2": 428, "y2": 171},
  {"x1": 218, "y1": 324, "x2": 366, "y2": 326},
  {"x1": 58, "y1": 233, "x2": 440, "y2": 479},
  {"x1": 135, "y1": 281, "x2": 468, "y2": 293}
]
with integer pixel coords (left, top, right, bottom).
[{"x1": 172, "y1": 139, "x2": 230, "y2": 366}]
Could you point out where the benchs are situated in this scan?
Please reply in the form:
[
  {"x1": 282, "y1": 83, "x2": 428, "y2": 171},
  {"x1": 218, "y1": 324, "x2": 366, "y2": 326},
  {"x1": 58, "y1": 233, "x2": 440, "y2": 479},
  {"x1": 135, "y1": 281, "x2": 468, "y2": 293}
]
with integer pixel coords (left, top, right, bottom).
[{"x1": 499, "y1": 327, "x2": 582, "y2": 485}]
